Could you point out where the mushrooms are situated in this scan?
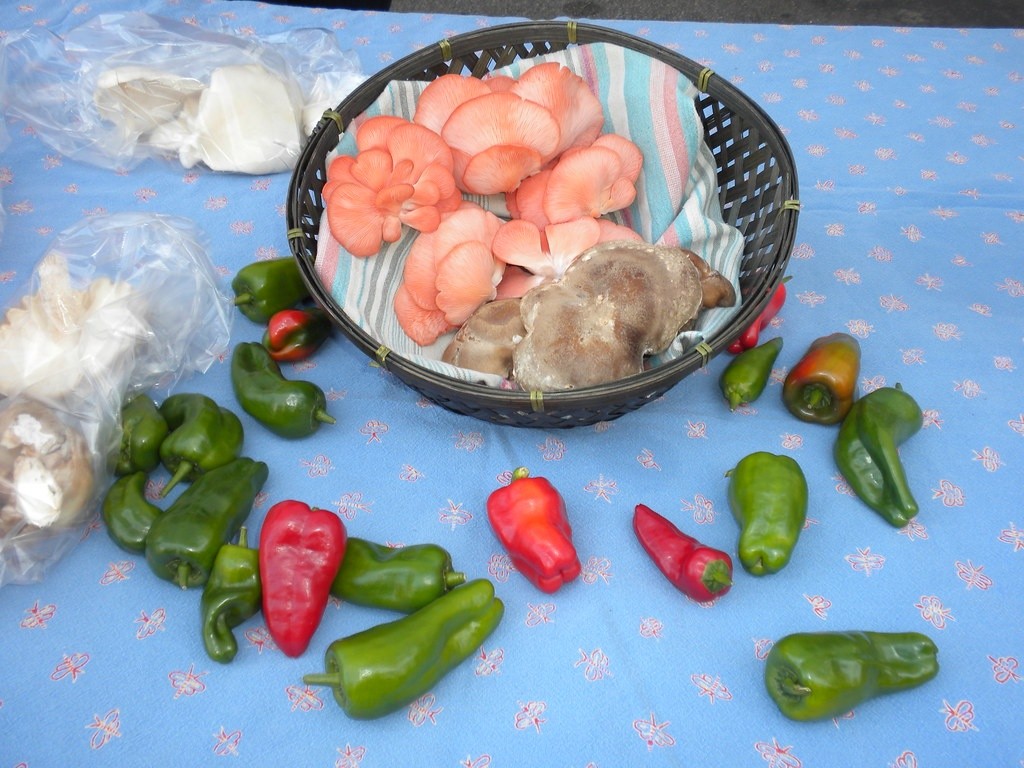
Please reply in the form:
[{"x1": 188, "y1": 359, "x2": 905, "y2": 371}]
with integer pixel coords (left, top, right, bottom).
[
  {"x1": 2, "y1": 61, "x2": 364, "y2": 530},
  {"x1": 324, "y1": 63, "x2": 734, "y2": 396}
]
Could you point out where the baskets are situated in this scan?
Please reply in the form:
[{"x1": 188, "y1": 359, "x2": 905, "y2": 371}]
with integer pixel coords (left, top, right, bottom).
[{"x1": 283, "y1": 25, "x2": 803, "y2": 428}]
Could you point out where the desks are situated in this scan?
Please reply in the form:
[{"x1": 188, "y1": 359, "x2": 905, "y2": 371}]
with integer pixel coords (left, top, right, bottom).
[{"x1": 1, "y1": 2, "x2": 1021, "y2": 764}]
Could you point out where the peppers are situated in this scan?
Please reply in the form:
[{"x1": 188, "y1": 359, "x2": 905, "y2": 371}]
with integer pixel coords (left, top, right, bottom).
[{"x1": 105, "y1": 255, "x2": 938, "y2": 722}]
